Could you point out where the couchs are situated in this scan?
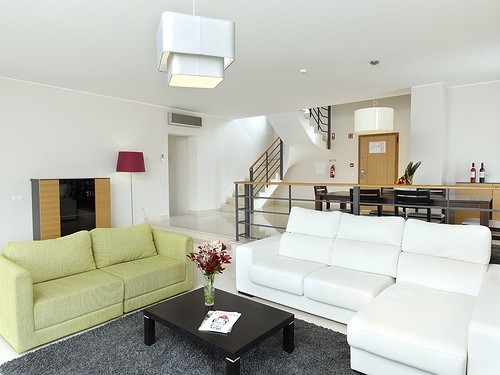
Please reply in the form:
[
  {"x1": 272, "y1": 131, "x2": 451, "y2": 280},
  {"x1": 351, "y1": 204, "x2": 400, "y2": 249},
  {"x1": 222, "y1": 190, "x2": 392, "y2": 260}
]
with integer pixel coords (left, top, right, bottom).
[
  {"x1": 235, "y1": 206, "x2": 500, "y2": 375},
  {"x1": 0, "y1": 222, "x2": 195, "y2": 355}
]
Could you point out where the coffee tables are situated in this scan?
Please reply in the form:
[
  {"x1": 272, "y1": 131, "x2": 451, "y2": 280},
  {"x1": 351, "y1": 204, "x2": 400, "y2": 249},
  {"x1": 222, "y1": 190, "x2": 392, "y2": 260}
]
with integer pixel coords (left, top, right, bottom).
[{"x1": 141, "y1": 285, "x2": 296, "y2": 375}]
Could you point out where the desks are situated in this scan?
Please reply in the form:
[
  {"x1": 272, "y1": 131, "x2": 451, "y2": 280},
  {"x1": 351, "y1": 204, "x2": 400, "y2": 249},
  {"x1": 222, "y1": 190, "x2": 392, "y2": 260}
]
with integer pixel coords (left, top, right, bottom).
[{"x1": 314, "y1": 189, "x2": 493, "y2": 226}]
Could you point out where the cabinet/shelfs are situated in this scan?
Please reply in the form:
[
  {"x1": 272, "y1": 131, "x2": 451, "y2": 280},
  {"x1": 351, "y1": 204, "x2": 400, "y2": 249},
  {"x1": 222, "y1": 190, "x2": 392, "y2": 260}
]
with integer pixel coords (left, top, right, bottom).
[{"x1": 454, "y1": 181, "x2": 500, "y2": 225}]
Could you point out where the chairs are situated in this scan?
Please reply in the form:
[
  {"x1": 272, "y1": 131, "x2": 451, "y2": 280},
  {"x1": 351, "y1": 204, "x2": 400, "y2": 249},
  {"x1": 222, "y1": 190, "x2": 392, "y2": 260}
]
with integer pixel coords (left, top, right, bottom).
[
  {"x1": 348, "y1": 186, "x2": 383, "y2": 216},
  {"x1": 314, "y1": 186, "x2": 330, "y2": 194},
  {"x1": 461, "y1": 218, "x2": 500, "y2": 241},
  {"x1": 407, "y1": 187, "x2": 447, "y2": 219},
  {"x1": 369, "y1": 186, "x2": 395, "y2": 215},
  {"x1": 393, "y1": 188, "x2": 441, "y2": 223}
]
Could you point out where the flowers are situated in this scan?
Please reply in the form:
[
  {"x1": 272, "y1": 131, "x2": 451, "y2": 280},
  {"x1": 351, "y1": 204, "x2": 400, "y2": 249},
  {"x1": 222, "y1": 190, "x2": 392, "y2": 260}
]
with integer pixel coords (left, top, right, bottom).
[{"x1": 186, "y1": 240, "x2": 233, "y2": 303}]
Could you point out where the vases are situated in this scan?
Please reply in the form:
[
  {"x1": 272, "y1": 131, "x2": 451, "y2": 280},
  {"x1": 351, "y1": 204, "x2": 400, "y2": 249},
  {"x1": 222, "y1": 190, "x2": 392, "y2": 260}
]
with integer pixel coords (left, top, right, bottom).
[{"x1": 202, "y1": 273, "x2": 216, "y2": 306}]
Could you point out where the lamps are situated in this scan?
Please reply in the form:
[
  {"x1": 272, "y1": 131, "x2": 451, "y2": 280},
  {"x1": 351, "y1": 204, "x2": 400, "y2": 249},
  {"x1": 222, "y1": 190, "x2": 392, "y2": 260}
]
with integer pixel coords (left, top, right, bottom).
[
  {"x1": 354, "y1": 60, "x2": 395, "y2": 135},
  {"x1": 156, "y1": 0, "x2": 238, "y2": 90},
  {"x1": 115, "y1": 148, "x2": 146, "y2": 224}
]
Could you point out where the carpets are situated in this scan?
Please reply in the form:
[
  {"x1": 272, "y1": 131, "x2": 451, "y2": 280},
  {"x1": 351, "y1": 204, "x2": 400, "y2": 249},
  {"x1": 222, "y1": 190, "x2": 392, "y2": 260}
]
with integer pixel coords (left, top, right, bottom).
[{"x1": 0, "y1": 311, "x2": 364, "y2": 375}]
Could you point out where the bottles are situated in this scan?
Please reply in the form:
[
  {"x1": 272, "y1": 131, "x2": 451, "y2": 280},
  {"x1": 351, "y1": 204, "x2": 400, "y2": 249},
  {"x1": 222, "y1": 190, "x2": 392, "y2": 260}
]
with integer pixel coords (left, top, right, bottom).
[
  {"x1": 471, "y1": 163, "x2": 476, "y2": 183},
  {"x1": 479, "y1": 162, "x2": 485, "y2": 183}
]
